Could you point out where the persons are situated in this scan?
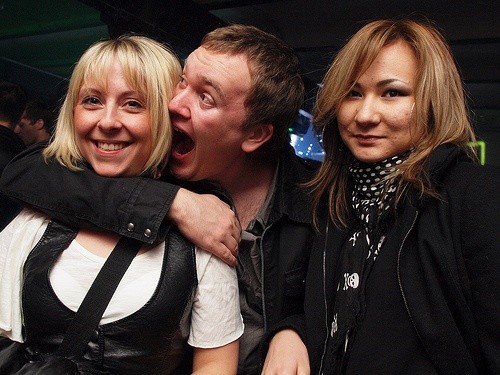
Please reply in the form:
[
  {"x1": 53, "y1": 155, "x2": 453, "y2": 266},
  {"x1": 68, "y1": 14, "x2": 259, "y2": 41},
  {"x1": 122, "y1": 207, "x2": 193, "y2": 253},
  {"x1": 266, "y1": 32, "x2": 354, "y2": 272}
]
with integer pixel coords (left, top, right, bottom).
[
  {"x1": 261, "y1": 18, "x2": 499, "y2": 375},
  {"x1": 16, "y1": 103, "x2": 54, "y2": 146},
  {"x1": 0, "y1": 35, "x2": 245, "y2": 374},
  {"x1": 0, "y1": 23, "x2": 322, "y2": 375},
  {"x1": 0, "y1": 81, "x2": 29, "y2": 233}
]
